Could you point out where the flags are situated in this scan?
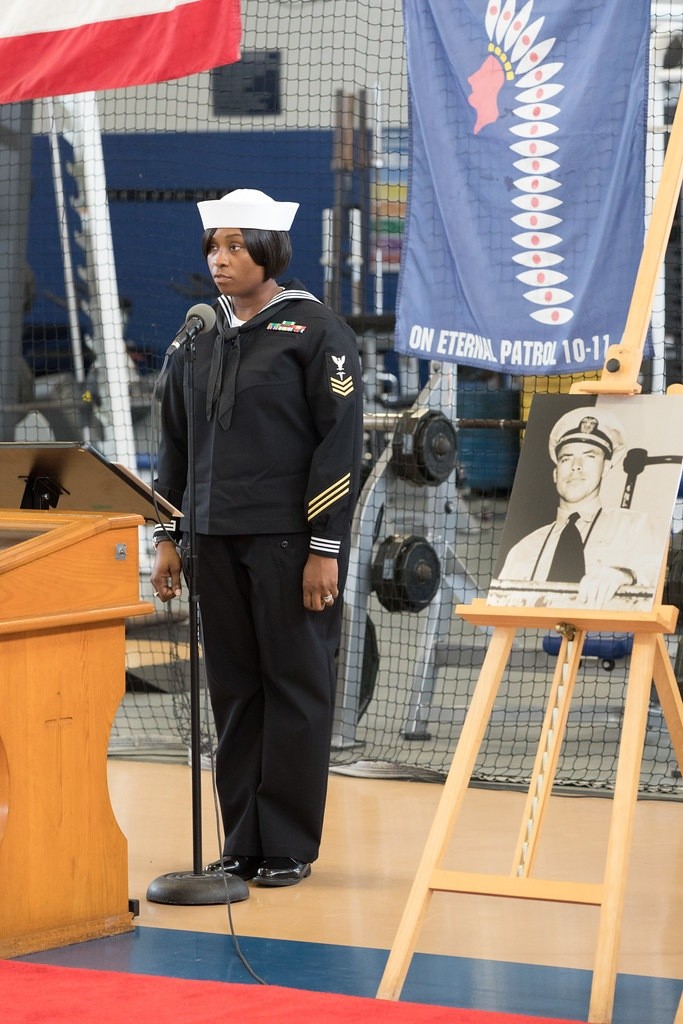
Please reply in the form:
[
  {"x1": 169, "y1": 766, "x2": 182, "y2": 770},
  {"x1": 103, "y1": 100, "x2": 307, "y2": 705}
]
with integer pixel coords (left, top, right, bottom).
[
  {"x1": 0, "y1": 0, "x2": 242, "y2": 103},
  {"x1": 392, "y1": 0, "x2": 655, "y2": 375}
]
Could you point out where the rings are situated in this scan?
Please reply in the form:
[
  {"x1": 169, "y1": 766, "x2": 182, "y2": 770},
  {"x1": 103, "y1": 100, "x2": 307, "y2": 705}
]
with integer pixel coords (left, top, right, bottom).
[
  {"x1": 322, "y1": 594, "x2": 333, "y2": 603},
  {"x1": 153, "y1": 591, "x2": 160, "y2": 597}
]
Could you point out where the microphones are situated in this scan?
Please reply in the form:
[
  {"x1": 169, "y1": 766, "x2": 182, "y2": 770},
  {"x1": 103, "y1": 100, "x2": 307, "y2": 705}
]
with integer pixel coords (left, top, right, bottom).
[{"x1": 164, "y1": 304, "x2": 217, "y2": 355}]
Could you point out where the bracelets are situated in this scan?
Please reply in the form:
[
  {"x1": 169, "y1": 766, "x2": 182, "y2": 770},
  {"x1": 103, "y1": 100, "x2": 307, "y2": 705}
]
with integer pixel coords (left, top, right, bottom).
[{"x1": 614, "y1": 566, "x2": 637, "y2": 586}]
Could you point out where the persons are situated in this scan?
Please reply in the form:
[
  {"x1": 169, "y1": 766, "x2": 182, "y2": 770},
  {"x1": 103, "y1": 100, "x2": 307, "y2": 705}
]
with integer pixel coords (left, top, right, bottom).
[
  {"x1": 497, "y1": 406, "x2": 664, "y2": 608},
  {"x1": 151, "y1": 189, "x2": 365, "y2": 886}
]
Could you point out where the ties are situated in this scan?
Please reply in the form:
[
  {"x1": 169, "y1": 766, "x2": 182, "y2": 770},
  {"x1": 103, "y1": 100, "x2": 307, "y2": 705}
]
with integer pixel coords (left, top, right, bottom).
[
  {"x1": 546, "y1": 511, "x2": 585, "y2": 583},
  {"x1": 205, "y1": 300, "x2": 292, "y2": 429}
]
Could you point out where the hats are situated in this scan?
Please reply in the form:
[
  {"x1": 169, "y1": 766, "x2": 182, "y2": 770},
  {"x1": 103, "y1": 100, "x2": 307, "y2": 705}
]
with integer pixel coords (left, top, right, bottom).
[
  {"x1": 195, "y1": 187, "x2": 301, "y2": 232},
  {"x1": 547, "y1": 405, "x2": 630, "y2": 471}
]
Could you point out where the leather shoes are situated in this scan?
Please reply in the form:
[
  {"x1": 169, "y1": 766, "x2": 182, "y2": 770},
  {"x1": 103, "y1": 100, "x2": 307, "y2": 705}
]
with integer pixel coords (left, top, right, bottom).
[
  {"x1": 204, "y1": 855, "x2": 261, "y2": 881},
  {"x1": 252, "y1": 856, "x2": 312, "y2": 885}
]
